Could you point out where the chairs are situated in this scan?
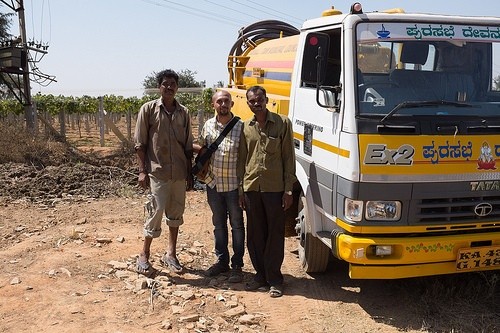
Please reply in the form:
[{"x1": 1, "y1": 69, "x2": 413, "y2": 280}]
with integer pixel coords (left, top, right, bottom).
[{"x1": 392, "y1": 42, "x2": 439, "y2": 103}]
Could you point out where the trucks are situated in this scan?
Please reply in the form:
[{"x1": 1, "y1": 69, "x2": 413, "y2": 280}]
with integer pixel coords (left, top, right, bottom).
[{"x1": 214, "y1": 4, "x2": 500, "y2": 283}]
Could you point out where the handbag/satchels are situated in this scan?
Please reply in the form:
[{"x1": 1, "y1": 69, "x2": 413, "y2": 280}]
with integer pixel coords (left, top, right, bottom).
[{"x1": 192, "y1": 145, "x2": 211, "y2": 181}]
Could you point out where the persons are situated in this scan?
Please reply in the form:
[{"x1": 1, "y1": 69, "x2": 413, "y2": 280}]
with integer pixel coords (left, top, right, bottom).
[
  {"x1": 236, "y1": 86, "x2": 296, "y2": 298},
  {"x1": 134, "y1": 69, "x2": 193, "y2": 275},
  {"x1": 195, "y1": 92, "x2": 248, "y2": 285}
]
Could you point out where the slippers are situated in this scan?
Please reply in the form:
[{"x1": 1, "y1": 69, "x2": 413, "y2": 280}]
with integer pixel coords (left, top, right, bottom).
[
  {"x1": 161, "y1": 251, "x2": 184, "y2": 274},
  {"x1": 135, "y1": 257, "x2": 152, "y2": 276}
]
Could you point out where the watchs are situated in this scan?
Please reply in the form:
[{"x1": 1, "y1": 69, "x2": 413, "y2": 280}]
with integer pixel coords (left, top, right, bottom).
[{"x1": 284, "y1": 191, "x2": 292, "y2": 196}]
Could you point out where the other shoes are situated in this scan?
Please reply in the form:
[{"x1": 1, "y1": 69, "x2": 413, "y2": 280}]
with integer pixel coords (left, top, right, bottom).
[
  {"x1": 244, "y1": 273, "x2": 267, "y2": 291},
  {"x1": 269, "y1": 283, "x2": 283, "y2": 297},
  {"x1": 228, "y1": 265, "x2": 242, "y2": 282},
  {"x1": 204, "y1": 262, "x2": 230, "y2": 276}
]
